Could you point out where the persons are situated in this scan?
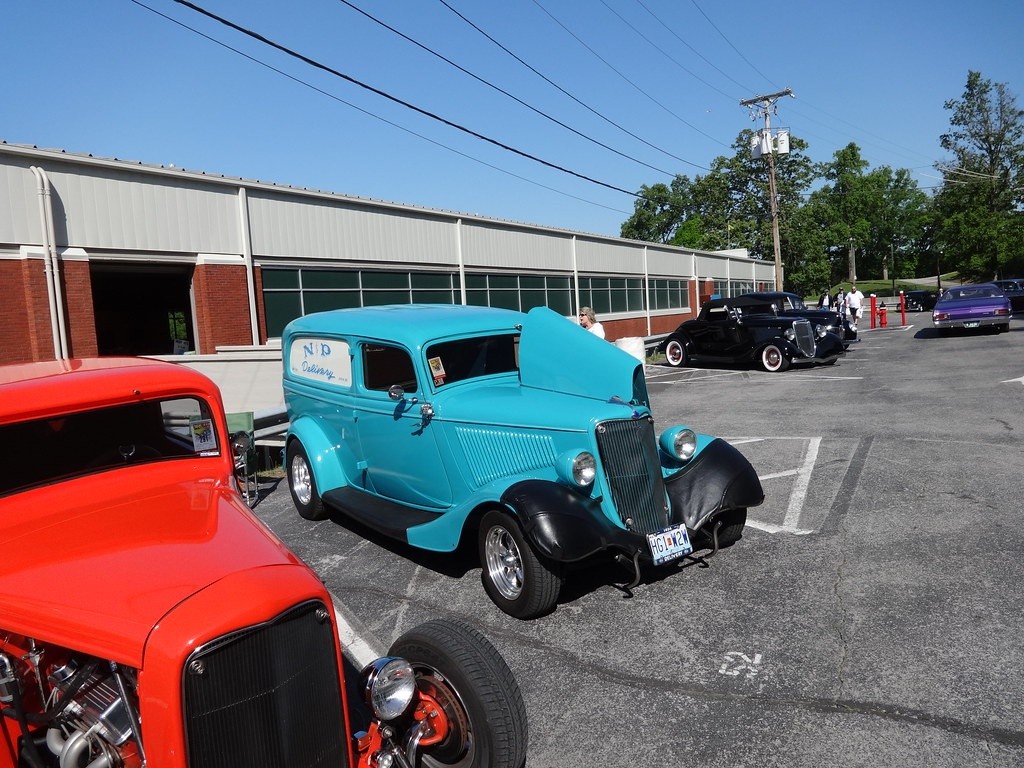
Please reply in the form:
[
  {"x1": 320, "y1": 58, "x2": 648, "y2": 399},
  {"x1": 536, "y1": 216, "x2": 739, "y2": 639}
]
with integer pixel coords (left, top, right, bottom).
[
  {"x1": 579, "y1": 307, "x2": 605, "y2": 339},
  {"x1": 845, "y1": 286, "x2": 864, "y2": 324},
  {"x1": 833, "y1": 287, "x2": 847, "y2": 313},
  {"x1": 818, "y1": 289, "x2": 833, "y2": 313}
]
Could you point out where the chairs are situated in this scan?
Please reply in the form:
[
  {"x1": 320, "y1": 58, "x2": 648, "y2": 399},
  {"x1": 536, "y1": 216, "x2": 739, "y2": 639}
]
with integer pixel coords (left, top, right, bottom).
[{"x1": 226, "y1": 412, "x2": 259, "y2": 509}]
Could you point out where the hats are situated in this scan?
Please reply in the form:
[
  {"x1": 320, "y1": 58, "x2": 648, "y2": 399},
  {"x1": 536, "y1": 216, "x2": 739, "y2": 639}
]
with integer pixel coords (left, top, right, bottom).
[{"x1": 839, "y1": 287, "x2": 843, "y2": 290}]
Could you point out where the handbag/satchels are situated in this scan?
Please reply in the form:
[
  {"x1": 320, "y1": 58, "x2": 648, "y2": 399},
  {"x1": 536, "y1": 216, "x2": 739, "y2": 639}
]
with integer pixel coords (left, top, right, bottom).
[{"x1": 856, "y1": 308, "x2": 863, "y2": 318}]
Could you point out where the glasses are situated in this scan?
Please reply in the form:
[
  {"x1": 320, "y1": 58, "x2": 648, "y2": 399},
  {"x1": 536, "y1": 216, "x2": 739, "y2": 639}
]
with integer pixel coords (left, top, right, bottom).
[{"x1": 579, "y1": 313, "x2": 586, "y2": 317}]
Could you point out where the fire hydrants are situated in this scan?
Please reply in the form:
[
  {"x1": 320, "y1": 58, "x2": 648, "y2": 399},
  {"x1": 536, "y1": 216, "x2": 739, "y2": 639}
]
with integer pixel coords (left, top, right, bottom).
[{"x1": 878, "y1": 299, "x2": 889, "y2": 328}]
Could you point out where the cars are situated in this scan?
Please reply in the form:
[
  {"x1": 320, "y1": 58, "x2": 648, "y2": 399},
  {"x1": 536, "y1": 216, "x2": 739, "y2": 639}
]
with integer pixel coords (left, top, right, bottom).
[
  {"x1": 0, "y1": 360, "x2": 530, "y2": 768},
  {"x1": 991, "y1": 278, "x2": 1024, "y2": 303},
  {"x1": 896, "y1": 289, "x2": 947, "y2": 315},
  {"x1": 279, "y1": 301, "x2": 759, "y2": 622},
  {"x1": 661, "y1": 290, "x2": 859, "y2": 372},
  {"x1": 932, "y1": 283, "x2": 1013, "y2": 332}
]
